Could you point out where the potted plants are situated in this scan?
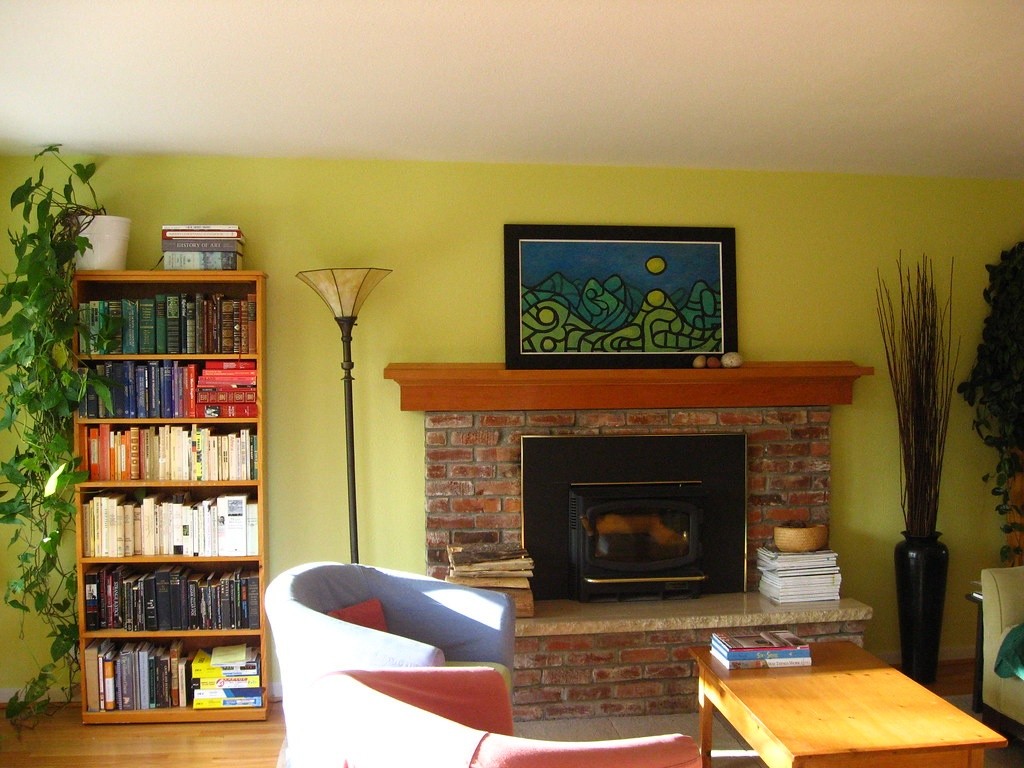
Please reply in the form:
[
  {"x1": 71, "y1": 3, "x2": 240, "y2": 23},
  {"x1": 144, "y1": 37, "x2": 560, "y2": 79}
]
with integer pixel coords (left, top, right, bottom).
[
  {"x1": 0, "y1": 144, "x2": 132, "y2": 737},
  {"x1": 957, "y1": 240, "x2": 1024, "y2": 566}
]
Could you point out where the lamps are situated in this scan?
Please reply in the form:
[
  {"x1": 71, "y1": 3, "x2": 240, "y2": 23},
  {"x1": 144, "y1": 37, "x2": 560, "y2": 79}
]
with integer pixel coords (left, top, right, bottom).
[{"x1": 296, "y1": 267, "x2": 392, "y2": 564}]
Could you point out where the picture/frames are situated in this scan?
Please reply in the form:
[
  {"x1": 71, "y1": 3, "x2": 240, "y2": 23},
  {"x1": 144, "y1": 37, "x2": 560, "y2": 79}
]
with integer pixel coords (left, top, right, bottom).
[{"x1": 504, "y1": 224, "x2": 738, "y2": 370}]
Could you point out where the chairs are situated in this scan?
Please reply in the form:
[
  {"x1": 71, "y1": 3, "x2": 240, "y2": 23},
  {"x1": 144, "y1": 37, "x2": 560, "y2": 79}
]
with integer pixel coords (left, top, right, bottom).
[
  {"x1": 263, "y1": 562, "x2": 516, "y2": 711},
  {"x1": 286, "y1": 666, "x2": 702, "y2": 768},
  {"x1": 981, "y1": 567, "x2": 1024, "y2": 745}
]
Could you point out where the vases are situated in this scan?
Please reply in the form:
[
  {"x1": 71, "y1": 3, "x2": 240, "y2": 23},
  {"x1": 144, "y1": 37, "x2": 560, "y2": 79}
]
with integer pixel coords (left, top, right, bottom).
[{"x1": 894, "y1": 531, "x2": 948, "y2": 685}]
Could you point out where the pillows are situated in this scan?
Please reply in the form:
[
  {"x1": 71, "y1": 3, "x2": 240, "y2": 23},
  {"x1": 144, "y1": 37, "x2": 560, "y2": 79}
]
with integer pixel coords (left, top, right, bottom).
[{"x1": 325, "y1": 598, "x2": 387, "y2": 632}]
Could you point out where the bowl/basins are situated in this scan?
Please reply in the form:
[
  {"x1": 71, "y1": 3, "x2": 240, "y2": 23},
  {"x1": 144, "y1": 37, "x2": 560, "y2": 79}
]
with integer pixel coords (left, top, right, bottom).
[{"x1": 774, "y1": 523, "x2": 827, "y2": 552}]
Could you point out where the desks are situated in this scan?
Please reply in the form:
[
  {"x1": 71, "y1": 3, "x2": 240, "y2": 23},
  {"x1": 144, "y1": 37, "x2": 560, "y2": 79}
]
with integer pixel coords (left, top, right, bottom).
[{"x1": 687, "y1": 641, "x2": 1008, "y2": 768}]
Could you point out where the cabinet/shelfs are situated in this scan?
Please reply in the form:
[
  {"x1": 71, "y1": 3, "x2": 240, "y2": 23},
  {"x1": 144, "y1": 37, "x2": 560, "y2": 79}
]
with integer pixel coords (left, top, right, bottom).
[{"x1": 71, "y1": 269, "x2": 271, "y2": 724}]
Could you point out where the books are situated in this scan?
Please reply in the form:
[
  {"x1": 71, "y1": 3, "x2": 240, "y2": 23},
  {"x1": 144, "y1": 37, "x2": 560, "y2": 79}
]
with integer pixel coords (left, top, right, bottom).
[
  {"x1": 85, "y1": 639, "x2": 262, "y2": 712},
  {"x1": 709, "y1": 630, "x2": 811, "y2": 669},
  {"x1": 88, "y1": 423, "x2": 257, "y2": 482},
  {"x1": 83, "y1": 493, "x2": 259, "y2": 556},
  {"x1": 79, "y1": 360, "x2": 257, "y2": 419},
  {"x1": 79, "y1": 294, "x2": 257, "y2": 354},
  {"x1": 757, "y1": 546, "x2": 843, "y2": 603},
  {"x1": 85, "y1": 564, "x2": 259, "y2": 631},
  {"x1": 162, "y1": 224, "x2": 247, "y2": 270}
]
